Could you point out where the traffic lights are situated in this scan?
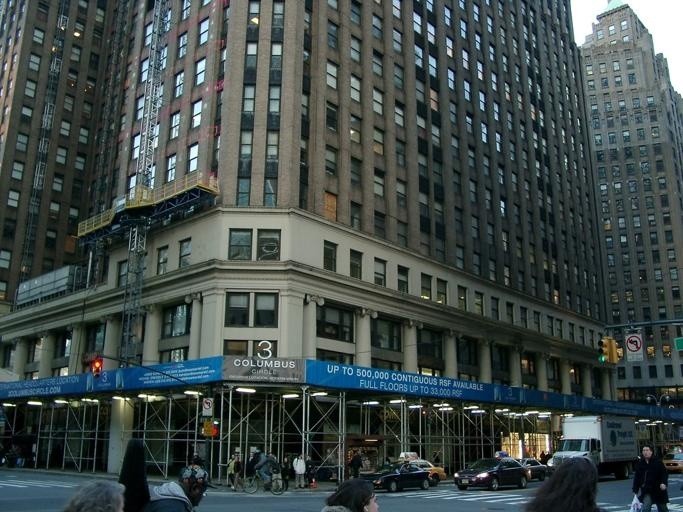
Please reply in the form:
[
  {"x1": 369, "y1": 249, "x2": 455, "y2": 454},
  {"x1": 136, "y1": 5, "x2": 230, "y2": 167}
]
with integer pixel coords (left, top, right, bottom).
[
  {"x1": 92, "y1": 359, "x2": 100, "y2": 380},
  {"x1": 597, "y1": 336, "x2": 612, "y2": 363},
  {"x1": 610, "y1": 340, "x2": 620, "y2": 364},
  {"x1": 211, "y1": 423, "x2": 220, "y2": 437}
]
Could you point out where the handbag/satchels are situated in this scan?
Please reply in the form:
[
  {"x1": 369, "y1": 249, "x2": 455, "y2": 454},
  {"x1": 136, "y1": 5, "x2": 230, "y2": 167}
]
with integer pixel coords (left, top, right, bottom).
[{"x1": 638, "y1": 486, "x2": 643, "y2": 503}]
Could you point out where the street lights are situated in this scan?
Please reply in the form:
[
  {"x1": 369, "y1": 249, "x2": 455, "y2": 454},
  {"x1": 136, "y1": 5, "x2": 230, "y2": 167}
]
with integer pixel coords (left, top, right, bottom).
[
  {"x1": 309, "y1": 477, "x2": 316, "y2": 489},
  {"x1": 645, "y1": 393, "x2": 670, "y2": 456}
]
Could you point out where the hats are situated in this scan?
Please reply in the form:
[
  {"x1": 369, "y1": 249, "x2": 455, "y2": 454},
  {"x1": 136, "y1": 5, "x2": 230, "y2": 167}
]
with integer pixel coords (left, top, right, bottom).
[{"x1": 180, "y1": 463, "x2": 217, "y2": 489}]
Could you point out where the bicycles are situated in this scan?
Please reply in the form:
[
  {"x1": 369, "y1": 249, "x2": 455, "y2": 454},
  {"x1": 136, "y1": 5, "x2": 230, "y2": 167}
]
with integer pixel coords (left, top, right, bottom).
[{"x1": 241, "y1": 466, "x2": 286, "y2": 497}]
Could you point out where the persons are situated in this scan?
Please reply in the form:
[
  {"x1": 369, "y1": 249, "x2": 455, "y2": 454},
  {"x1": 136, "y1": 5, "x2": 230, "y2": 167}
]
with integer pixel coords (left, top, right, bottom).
[
  {"x1": 63, "y1": 480, "x2": 126, "y2": 512},
  {"x1": 523, "y1": 453, "x2": 609, "y2": 512},
  {"x1": 540, "y1": 451, "x2": 546, "y2": 463},
  {"x1": 631, "y1": 445, "x2": 669, "y2": 512},
  {"x1": 319, "y1": 479, "x2": 380, "y2": 512},
  {"x1": 347, "y1": 451, "x2": 363, "y2": 479},
  {"x1": 225, "y1": 450, "x2": 317, "y2": 492},
  {"x1": 148, "y1": 464, "x2": 209, "y2": 512}
]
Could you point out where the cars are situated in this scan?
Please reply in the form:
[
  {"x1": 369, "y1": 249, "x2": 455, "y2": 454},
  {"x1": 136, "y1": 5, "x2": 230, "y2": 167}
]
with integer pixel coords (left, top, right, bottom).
[
  {"x1": 361, "y1": 464, "x2": 432, "y2": 493},
  {"x1": 515, "y1": 458, "x2": 547, "y2": 481},
  {"x1": 661, "y1": 451, "x2": 682, "y2": 473},
  {"x1": 453, "y1": 451, "x2": 531, "y2": 489},
  {"x1": 392, "y1": 452, "x2": 449, "y2": 487}
]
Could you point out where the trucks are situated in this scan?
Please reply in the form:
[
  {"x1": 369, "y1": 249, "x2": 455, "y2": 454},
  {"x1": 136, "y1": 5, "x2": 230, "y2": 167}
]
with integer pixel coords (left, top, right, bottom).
[{"x1": 545, "y1": 414, "x2": 641, "y2": 483}]
